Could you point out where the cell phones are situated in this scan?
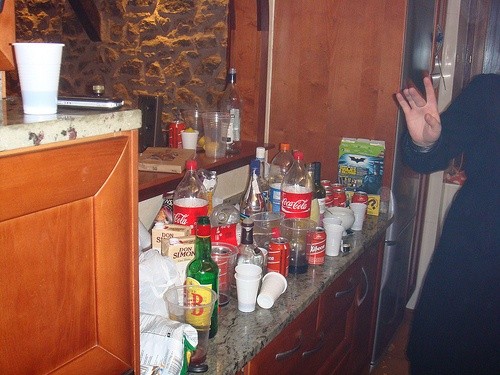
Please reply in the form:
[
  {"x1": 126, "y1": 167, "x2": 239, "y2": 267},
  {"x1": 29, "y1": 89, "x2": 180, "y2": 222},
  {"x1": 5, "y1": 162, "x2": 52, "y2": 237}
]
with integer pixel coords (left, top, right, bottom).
[{"x1": 56, "y1": 96, "x2": 125, "y2": 111}]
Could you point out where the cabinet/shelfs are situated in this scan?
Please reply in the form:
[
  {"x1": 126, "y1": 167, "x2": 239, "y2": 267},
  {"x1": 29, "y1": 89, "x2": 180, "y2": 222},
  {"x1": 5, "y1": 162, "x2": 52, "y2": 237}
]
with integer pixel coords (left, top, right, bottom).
[
  {"x1": 269, "y1": 0, "x2": 448, "y2": 375},
  {"x1": 244, "y1": 232, "x2": 386, "y2": 375},
  {"x1": 0, "y1": 129, "x2": 143, "y2": 375}
]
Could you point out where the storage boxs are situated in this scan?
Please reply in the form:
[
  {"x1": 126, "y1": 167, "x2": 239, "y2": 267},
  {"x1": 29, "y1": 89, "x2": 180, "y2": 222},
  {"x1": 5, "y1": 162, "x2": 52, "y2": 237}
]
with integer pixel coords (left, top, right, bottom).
[{"x1": 337, "y1": 137, "x2": 385, "y2": 216}]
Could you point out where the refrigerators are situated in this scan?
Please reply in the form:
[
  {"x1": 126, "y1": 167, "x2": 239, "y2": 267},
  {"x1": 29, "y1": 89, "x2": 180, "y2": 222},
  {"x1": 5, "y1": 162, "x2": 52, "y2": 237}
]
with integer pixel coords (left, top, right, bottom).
[{"x1": 372, "y1": 0, "x2": 435, "y2": 369}]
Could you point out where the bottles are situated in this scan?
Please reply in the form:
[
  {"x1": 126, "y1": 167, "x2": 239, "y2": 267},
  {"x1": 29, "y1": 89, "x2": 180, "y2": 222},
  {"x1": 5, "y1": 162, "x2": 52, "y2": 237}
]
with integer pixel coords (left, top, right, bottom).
[
  {"x1": 173, "y1": 160, "x2": 209, "y2": 235},
  {"x1": 185, "y1": 216, "x2": 218, "y2": 338},
  {"x1": 281, "y1": 152, "x2": 312, "y2": 219},
  {"x1": 305, "y1": 163, "x2": 326, "y2": 226},
  {"x1": 230, "y1": 220, "x2": 265, "y2": 289},
  {"x1": 217, "y1": 68, "x2": 241, "y2": 153},
  {"x1": 268, "y1": 144, "x2": 293, "y2": 213},
  {"x1": 240, "y1": 146, "x2": 269, "y2": 223}
]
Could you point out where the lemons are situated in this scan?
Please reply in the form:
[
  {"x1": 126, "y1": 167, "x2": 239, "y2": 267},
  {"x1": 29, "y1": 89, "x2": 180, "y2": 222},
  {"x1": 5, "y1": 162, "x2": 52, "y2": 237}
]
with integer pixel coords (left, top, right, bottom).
[
  {"x1": 184, "y1": 127, "x2": 195, "y2": 133},
  {"x1": 198, "y1": 136, "x2": 218, "y2": 152}
]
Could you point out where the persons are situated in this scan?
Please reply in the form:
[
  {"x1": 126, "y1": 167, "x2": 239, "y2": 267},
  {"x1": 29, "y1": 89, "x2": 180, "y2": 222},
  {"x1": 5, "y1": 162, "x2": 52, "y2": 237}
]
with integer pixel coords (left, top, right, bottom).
[{"x1": 396, "y1": 74, "x2": 500, "y2": 375}]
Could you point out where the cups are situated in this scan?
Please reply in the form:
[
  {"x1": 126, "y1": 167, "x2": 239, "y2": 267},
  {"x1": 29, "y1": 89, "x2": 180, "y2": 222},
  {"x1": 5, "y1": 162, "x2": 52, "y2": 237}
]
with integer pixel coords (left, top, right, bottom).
[
  {"x1": 12, "y1": 42, "x2": 65, "y2": 114},
  {"x1": 210, "y1": 242, "x2": 239, "y2": 307},
  {"x1": 234, "y1": 264, "x2": 262, "y2": 312},
  {"x1": 164, "y1": 285, "x2": 217, "y2": 364},
  {"x1": 323, "y1": 218, "x2": 341, "y2": 229},
  {"x1": 258, "y1": 247, "x2": 268, "y2": 277},
  {"x1": 181, "y1": 130, "x2": 199, "y2": 159},
  {"x1": 324, "y1": 225, "x2": 344, "y2": 257},
  {"x1": 250, "y1": 212, "x2": 317, "y2": 273},
  {"x1": 257, "y1": 271, "x2": 287, "y2": 309},
  {"x1": 183, "y1": 111, "x2": 205, "y2": 150},
  {"x1": 200, "y1": 112, "x2": 230, "y2": 158},
  {"x1": 349, "y1": 203, "x2": 366, "y2": 231}
]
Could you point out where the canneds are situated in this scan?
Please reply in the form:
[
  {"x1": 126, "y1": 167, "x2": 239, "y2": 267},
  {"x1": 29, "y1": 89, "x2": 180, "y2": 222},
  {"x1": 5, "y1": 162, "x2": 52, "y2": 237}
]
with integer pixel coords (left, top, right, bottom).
[
  {"x1": 168, "y1": 120, "x2": 186, "y2": 148},
  {"x1": 267, "y1": 237, "x2": 289, "y2": 278},
  {"x1": 210, "y1": 247, "x2": 231, "y2": 291},
  {"x1": 320, "y1": 179, "x2": 368, "y2": 221},
  {"x1": 305, "y1": 227, "x2": 326, "y2": 265}
]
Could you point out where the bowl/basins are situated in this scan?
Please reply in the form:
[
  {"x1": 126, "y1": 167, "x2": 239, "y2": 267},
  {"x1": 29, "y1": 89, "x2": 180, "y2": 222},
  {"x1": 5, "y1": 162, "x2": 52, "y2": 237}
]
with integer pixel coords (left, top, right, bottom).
[{"x1": 325, "y1": 207, "x2": 354, "y2": 236}]
